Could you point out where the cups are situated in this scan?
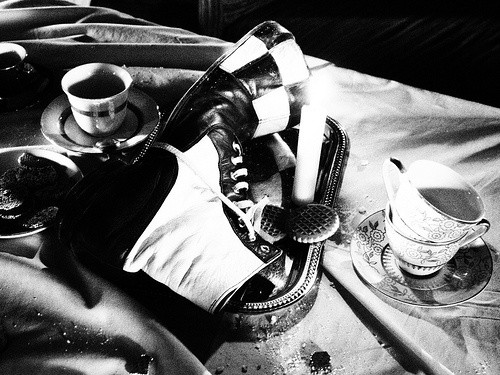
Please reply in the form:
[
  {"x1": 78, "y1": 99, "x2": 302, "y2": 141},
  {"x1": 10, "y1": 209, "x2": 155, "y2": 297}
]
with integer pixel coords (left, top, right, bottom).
[
  {"x1": 61, "y1": 63, "x2": 133, "y2": 136},
  {"x1": 383, "y1": 155, "x2": 486, "y2": 246},
  {"x1": 385, "y1": 203, "x2": 491, "y2": 278},
  {"x1": 0, "y1": 40, "x2": 35, "y2": 98}
]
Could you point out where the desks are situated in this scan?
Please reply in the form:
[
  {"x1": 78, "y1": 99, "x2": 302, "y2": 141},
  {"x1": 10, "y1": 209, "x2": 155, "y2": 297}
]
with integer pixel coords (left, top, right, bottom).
[{"x1": 0, "y1": 0, "x2": 500, "y2": 375}]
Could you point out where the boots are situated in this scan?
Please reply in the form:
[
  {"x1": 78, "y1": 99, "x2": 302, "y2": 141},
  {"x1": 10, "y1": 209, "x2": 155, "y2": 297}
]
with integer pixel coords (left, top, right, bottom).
[{"x1": 47, "y1": 20, "x2": 310, "y2": 318}]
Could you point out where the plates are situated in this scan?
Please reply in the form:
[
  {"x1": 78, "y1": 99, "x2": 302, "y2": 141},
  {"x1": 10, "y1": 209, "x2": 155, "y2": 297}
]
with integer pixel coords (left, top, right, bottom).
[
  {"x1": 348, "y1": 208, "x2": 493, "y2": 311},
  {"x1": 1, "y1": 148, "x2": 85, "y2": 241},
  {"x1": 39, "y1": 89, "x2": 162, "y2": 155}
]
